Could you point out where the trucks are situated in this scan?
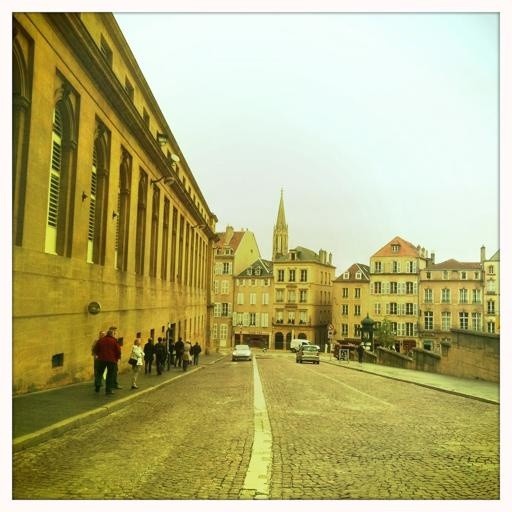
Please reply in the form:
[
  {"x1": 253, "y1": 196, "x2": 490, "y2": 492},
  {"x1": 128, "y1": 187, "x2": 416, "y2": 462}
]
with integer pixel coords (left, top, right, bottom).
[{"x1": 290, "y1": 339, "x2": 311, "y2": 353}]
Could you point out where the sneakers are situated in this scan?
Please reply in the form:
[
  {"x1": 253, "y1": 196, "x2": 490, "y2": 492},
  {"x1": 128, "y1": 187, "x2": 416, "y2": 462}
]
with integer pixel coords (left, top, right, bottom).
[
  {"x1": 95, "y1": 384, "x2": 122, "y2": 395},
  {"x1": 145, "y1": 363, "x2": 198, "y2": 376},
  {"x1": 132, "y1": 385, "x2": 139, "y2": 389}
]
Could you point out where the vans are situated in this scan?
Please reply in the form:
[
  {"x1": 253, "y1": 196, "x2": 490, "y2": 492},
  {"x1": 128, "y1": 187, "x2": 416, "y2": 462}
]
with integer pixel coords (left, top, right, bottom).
[{"x1": 295, "y1": 345, "x2": 320, "y2": 364}]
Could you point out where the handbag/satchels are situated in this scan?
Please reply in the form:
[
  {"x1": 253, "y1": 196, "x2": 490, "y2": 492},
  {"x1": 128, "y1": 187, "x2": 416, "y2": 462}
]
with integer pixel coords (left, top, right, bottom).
[{"x1": 128, "y1": 358, "x2": 137, "y2": 365}]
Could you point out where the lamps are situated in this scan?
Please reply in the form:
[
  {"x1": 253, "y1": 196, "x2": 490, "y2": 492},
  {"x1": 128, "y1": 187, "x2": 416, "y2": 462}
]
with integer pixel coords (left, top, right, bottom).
[
  {"x1": 191, "y1": 222, "x2": 206, "y2": 231},
  {"x1": 151, "y1": 174, "x2": 176, "y2": 186}
]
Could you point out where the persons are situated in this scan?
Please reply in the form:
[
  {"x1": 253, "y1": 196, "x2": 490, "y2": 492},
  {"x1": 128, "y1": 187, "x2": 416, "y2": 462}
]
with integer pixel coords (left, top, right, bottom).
[
  {"x1": 143, "y1": 336, "x2": 201, "y2": 375},
  {"x1": 92, "y1": 326, "x2": 123, "y2": 395},
  {"x1": 357, "y1": 345, "x2": 365, "y2": 362},
  {"x1": 130, "y1": 338, "x2": 146, "y2": 389}
]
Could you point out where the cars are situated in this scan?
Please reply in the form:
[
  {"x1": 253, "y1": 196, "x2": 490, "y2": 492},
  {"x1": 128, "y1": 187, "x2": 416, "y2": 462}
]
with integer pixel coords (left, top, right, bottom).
[{"x1": 231, "y1": 345, "x2": 252, "y2": 361}]
